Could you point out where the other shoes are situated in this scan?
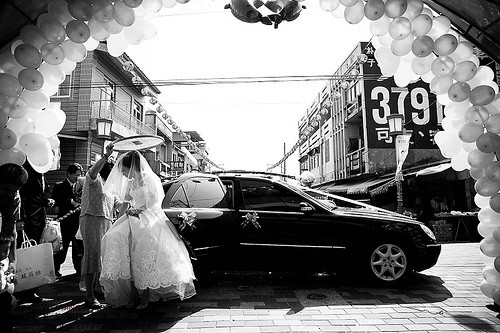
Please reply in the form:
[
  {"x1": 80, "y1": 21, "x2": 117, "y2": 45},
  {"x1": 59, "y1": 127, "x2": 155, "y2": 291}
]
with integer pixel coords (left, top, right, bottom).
[
  {"x1": 78, "y1": 283, "x2": 87, "y2": 292},
  {"x1": 85, "y1": 299, "x2": 104, "y2": 308}
]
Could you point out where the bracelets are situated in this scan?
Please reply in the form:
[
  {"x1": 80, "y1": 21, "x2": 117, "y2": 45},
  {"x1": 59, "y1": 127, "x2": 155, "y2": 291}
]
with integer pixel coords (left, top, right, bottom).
[{"x1": 103, "y1": 153, "x2": 108, "y2": 160}]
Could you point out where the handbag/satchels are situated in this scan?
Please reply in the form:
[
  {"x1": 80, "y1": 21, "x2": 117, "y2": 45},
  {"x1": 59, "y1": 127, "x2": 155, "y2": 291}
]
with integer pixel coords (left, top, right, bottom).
[
  {"x1": 14, "y1": 229, "x2": 57, "y2": 294},
  {"x1": 41, "y1": 219, "x2": 64, "y2": 254}
]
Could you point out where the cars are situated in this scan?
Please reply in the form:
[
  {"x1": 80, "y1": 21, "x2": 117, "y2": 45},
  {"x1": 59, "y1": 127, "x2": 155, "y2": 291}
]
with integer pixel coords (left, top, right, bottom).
[{"x1": 71, "y1": 173, "x2": 442, "y2": 288}]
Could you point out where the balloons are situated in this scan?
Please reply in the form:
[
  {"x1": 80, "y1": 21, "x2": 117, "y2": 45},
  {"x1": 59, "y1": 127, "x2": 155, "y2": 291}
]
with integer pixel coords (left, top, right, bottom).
[{"x1": 0, "y1": 0, "x2": 500, "y2": 307}]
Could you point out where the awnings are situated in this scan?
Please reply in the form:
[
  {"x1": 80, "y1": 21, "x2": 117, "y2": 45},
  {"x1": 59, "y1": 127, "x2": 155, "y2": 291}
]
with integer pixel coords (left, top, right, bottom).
[
  {"x1": 370, "y1": 179, "x2": 396, "y2": 196},
  {"x1": 347, "y1": 176, "x2": 394, "y2": 194},
  {"x1": 327, "y1": 182, "x2": 360, "y2": 193}
]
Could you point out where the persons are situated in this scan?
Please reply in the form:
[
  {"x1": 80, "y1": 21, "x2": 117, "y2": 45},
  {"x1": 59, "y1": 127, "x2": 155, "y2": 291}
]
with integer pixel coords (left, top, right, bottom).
[
  {"x1": 53, "y1": 163, "x2": 84, "y2": 278},
  {"x1": 102, "y1": 150, "x2": 165, "y2": 309},
  {"x1": 79, "y1": 144, "x2": 114, "y2": 308},
  {"x1": 0, "y1": 189, "x2": 21, "y2": 285},
  {"x1": 22, "y1": 171, "x2": 55, "y2": 301}
]
